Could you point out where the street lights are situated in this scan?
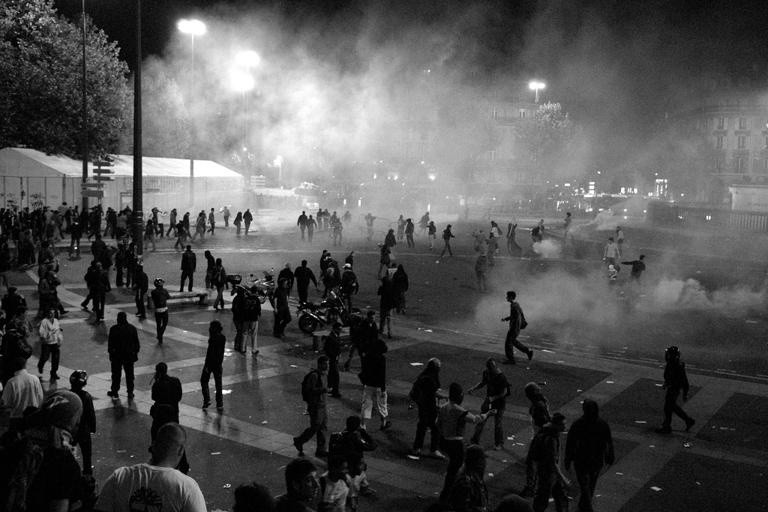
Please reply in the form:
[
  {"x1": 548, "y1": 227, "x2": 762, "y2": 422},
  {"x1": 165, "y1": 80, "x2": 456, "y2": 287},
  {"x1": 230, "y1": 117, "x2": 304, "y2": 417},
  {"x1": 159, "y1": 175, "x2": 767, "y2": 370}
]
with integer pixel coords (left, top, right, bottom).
[
  {"x1": 174, "y1": 18, "x2": 207, "y2": 207},
  {"x1": 529, "y1": 80, "x2": 546, "y2": 103},
  {"x1": 227, "y1": 45, "x2": 262, "y2": 208}
]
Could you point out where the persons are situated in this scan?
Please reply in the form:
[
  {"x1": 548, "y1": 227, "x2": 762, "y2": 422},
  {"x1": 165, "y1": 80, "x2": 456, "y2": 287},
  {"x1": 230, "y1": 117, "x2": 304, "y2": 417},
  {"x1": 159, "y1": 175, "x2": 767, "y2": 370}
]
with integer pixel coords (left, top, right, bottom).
[{"x1": 0, "y1": 200, "x2": 696, "y2": 511}]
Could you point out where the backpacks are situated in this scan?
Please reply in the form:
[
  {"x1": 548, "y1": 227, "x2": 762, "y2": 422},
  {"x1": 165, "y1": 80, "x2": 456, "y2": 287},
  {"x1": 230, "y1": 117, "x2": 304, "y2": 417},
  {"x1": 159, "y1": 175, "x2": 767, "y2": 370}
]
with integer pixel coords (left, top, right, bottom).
[{"x1": 301, "y1": 369, "x2": 324, "y2": 403}]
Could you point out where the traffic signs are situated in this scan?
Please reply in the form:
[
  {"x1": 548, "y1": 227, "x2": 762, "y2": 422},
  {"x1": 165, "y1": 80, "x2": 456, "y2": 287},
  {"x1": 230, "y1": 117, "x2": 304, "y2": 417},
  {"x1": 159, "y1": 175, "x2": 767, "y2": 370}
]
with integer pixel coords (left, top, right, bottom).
[{"x1": 80, "y1": 160, "x2": 116, "y2": 198}]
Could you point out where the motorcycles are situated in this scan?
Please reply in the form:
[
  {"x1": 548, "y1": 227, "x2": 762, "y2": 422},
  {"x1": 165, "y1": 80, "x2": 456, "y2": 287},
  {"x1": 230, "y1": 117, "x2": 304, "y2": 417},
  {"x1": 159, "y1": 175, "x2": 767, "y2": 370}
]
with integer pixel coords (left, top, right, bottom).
[
  {"x1": 226, "y1": 268, "x2": 278, "y2": 313},
  {"x1": 295, "y1": 284, "x2": 363, "y2": 333}
]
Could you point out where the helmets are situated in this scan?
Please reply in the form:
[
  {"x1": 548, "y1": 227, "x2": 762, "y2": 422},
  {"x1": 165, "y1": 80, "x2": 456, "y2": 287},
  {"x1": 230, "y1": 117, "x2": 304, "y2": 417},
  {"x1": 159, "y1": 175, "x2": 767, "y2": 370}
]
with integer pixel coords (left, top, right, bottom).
[
  {"x1": 664, "y1": 345, "x2": 682, "y2": 361},
  {"x1": 153, "y1": 277, "x2": 164, "y2": 288}
]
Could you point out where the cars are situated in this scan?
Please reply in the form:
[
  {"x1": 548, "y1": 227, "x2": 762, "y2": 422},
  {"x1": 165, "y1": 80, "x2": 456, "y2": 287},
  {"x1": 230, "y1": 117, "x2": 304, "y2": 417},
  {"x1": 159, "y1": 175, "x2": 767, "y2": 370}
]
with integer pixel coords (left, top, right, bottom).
[{"x1": 293, "y1": 181, "x2": 328, "y2": 197}]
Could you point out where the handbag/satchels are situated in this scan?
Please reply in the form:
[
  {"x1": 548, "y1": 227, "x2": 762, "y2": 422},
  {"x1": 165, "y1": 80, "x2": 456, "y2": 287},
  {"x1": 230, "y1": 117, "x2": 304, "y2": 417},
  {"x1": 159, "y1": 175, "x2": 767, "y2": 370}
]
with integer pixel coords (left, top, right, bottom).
[{"x1": 519, "y1": 316, "x2": 528, "y2": 330}]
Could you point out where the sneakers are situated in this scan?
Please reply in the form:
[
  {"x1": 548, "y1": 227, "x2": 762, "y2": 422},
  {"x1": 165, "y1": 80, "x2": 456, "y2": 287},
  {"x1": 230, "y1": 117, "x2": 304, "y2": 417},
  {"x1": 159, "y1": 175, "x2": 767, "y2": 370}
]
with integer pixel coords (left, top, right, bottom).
[
  {"x1": 380, "y1": 420, "x2": 392, "y2": 431},
  {"x1": 407, "y1": 449, "x2": 422, "y2": 460},
  {"x1": 202, "y1": 402, "x2": 211, "y2": 412},
  {"x1": 215, "y1": 401, "x2": 223, "y2": 409},
  {"x1": 492, "y1": 444, "x2": 505, "y2": 451},
  {"x1": 527, "y1": 350, "x2": 533, "y2": 360},
  {"x1": 684, "y1": 417, "x2": 696, "y2": 431},
  {"x1": 293, "y1": 434, "x2": 305, "y2": 453},
  {"x1": 502, "y1": 359, "x2": 516, "y2": 364},
  {"x1": 377, "y1": 328, "x2": 395, "y2": 339},
  {"x1": 654, "y1": 424, "x2": 673, "y2": 434},
  {"x1": 174, "y1": 281, "x2": 292, "y2": 355},
  {"x1": 37, "y1": 301, "x2": 169, "y2": 400},
  {"x1": 313, "y1": 450, "x2": 329, "y2": 458},
  {"x1": 430, "y1": 449, "x2": 446, "y2": 460}
]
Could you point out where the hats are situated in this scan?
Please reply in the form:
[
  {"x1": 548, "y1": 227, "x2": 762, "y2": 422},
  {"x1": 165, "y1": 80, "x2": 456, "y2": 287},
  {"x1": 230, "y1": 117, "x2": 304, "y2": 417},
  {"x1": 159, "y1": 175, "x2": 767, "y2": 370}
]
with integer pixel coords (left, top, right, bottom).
[
  {"x1": 464, "y1": 444, "x2": 490, "y2": 460},
  {"x1": 341, "y1": 262, "x2": 352, "y2": 269}
]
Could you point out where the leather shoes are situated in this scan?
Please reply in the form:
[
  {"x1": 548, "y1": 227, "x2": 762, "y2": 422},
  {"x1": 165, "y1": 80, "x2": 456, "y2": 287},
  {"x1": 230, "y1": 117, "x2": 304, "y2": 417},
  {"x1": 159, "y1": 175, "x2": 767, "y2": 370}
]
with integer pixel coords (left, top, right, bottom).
[{"x1": 80, "y1": 464, "x2": 96, "y2": 472}]
